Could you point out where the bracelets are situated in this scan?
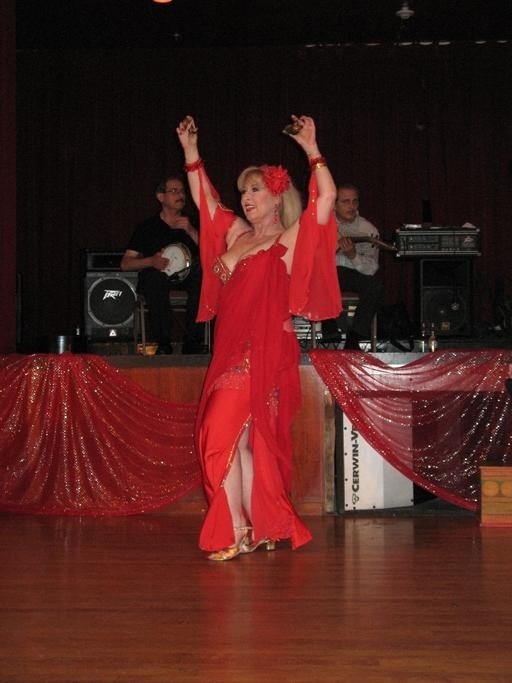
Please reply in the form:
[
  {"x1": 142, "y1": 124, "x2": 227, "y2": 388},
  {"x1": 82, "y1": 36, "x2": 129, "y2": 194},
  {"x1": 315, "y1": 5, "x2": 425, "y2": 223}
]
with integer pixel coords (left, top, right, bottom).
[
  {"x1": 309, "y1": 157, "x2": 328, "y2": 170},
  {"x1": 182, "y1": 158, "x2": 204, "y2": 172}
]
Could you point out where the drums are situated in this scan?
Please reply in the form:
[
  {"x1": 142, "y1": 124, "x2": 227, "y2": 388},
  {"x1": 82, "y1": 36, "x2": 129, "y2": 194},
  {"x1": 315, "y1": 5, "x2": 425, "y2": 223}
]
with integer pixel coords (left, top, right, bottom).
[{"x1": 157, "y1": 241, "x2": 200, "y2": 283}]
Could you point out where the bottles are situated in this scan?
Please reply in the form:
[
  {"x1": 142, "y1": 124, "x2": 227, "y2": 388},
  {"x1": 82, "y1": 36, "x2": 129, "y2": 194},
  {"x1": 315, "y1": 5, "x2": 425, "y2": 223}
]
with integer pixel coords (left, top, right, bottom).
[{"x1": 419, "y1": 322, "x2": 440, "y2": 353}]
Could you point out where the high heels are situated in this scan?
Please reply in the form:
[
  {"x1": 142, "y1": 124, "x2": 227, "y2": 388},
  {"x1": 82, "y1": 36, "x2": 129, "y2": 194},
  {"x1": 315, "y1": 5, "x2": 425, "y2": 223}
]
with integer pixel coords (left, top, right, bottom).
[
  {"x1": 239, "y1": 527, "x2": 279, "y2": 555},
  {"x1": 208, "y1": 526, "x2": 251, "y2": 561}
]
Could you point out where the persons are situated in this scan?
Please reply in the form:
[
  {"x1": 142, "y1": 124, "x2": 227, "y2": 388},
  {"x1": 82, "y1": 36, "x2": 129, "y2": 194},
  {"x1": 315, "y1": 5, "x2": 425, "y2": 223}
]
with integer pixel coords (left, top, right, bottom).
[
  {"x1": 119, "y1": 175, "x2": 208, "y2": 355},
  {"x1": 176, "y1": 114, "x2": 338, "y2": 561},
  {"x1": 321, "y1": 183, "x2": 385, "y2": 350}
]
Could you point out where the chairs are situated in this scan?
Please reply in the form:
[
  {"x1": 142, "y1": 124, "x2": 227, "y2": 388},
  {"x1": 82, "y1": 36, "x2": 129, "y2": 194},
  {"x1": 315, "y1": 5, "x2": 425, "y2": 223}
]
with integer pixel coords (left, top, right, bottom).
[
  {"x1": 134, "y1": 273, "x2": 211, "y2": 358},
  {"x1": 311, "y1": 291, "x2": 377, "y2": 353}
]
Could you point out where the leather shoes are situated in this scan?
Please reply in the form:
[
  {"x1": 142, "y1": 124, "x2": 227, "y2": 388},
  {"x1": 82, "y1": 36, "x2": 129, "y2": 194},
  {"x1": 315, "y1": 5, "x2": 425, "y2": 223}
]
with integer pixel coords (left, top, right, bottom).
[
  {"x1": 181, "y1": 344, "x2": 205, "y2": 354},
  {"x1": 155, "y1": 344, "x2": 173, "y2": 354},
  {"x1": 345, "y1": 327, "x2": 361, "y2": 349},
  {"x1": 321, "y1": 331, "x2": 342, "y2": 342}
]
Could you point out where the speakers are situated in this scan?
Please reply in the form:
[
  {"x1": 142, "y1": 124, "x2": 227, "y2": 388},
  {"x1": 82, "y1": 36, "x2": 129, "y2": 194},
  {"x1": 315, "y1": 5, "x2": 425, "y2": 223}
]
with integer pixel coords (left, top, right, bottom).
[
  {"x1": 84, "y1": 269, "x2": 141, "y2": 340},
  {"x1": 418, "y1": 253, "x2": 475, "y2": 339}
]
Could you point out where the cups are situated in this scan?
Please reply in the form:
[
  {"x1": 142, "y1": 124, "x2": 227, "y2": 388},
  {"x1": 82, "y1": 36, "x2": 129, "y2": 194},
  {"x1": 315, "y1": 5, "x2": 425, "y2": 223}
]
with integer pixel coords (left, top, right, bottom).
[{"x1": 54, "y1": 334, "x2": 73, "y2": 356}]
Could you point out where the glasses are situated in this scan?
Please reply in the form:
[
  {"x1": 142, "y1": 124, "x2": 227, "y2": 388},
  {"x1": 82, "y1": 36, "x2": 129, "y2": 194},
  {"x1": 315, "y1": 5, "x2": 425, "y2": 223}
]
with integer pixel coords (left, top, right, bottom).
[{"x1": 163, "y1": 187, "x2": 187, "y2": 195}]
